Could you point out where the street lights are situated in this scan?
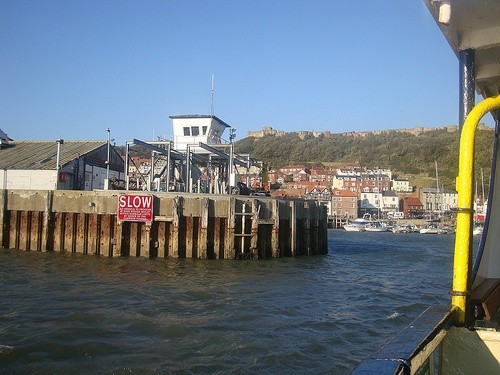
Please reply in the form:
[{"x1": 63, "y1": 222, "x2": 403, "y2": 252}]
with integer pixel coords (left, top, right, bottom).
[
  {"x1": 103, "y1": 128, "x2": 113, "y2": 190},
  {"x1": 54, "y1": 138, "x2": 65, "y2": 190}
]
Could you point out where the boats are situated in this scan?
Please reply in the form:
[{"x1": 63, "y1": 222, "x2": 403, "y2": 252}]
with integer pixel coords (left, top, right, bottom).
[
  {"x1": 390, "y1": 224, "x2": 419, "y2": 234},
  {"x1": 473, "y1": 225, "x2": 485, "y2": 235},
  {"x1": 418, "y1": 223, "x2": 450, "y2": 234},
  {"x1": 364, "y1": 221, "x2": 389, "y2": 232},
  {"x1": 342, "y1": 218, "x2": 370, "y2": 232}
]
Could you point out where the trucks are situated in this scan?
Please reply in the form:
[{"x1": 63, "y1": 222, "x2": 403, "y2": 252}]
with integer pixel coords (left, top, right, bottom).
[{"x1": 386, "y1": 210, "x2": 404, "y2": 219}]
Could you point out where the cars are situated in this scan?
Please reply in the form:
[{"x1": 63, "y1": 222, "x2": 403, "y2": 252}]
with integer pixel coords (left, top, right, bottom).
[
  {"x1": 237, "y1": 181, "x2": 270, "y2": 197},
  {"x1": 426, "y1": 217, "x2": 441, "y2": 222}
]
